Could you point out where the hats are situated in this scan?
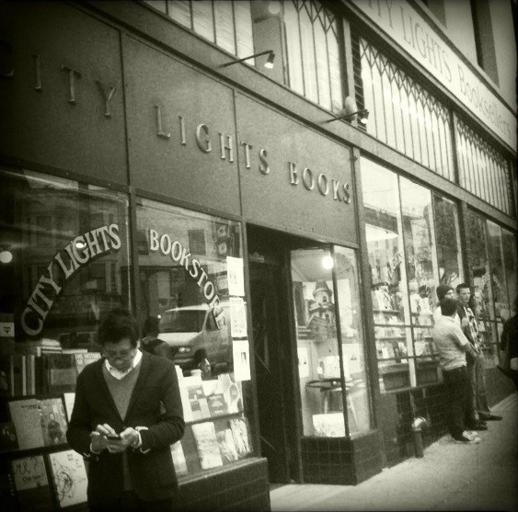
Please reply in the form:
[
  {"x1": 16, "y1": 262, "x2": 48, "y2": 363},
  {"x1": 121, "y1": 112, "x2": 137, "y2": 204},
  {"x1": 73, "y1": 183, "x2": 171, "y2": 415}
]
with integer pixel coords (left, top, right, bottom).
[{"x1": 436, "y1": 284, "x2": 453, "y2": 299}]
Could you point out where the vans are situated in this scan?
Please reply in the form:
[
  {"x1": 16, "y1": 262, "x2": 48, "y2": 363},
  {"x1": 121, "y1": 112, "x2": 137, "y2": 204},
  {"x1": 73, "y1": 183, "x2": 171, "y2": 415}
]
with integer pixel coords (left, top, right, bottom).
[{"x1": 156, "y1": 301, "x2": 233, "y2": 370}]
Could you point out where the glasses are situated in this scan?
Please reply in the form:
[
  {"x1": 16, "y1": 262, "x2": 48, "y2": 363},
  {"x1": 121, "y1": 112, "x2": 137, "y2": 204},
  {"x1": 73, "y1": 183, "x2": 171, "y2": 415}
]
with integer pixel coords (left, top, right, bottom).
[{"x1": 100, "y1": 348, "x2": 135, "y2": 361}]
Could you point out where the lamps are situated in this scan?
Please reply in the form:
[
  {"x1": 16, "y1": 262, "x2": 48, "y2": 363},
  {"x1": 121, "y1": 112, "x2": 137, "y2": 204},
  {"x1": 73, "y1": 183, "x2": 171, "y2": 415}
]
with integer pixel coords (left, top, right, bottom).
[
  {"x1": 326, "y1": 109, "x2": 369, "y2": 133},
  {"x1": 220, "y1": 50, "x2": 277, "y2": 72}
]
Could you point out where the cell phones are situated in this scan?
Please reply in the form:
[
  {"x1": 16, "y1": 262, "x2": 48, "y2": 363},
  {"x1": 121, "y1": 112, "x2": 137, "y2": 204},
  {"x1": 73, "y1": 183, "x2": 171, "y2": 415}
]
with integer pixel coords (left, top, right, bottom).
[{"x1": 107, "y1": 434, "x2": 122, "y2": 440}]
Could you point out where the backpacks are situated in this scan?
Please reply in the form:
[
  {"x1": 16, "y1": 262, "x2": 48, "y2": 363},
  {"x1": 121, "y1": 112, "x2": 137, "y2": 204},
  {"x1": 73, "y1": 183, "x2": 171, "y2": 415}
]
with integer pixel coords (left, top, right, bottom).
[{"x1": 138, "y1": 336, "x2": 162, "y2": 355}]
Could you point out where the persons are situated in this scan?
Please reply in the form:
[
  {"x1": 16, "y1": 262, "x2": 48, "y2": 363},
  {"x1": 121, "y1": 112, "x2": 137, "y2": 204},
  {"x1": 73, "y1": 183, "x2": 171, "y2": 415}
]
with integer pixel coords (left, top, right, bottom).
[
  {"x1": 137, "y1": 316, "x2": 174, "y2": 363},
  {"x1": 66, "y1": 307, "x2": 185, "y2": 512},
  {"x1": 499, "y1": 296, "x2": 518, "y2": 383},
  {"x1": 455, "y1": 283, "x2": 503, "y2": 425},
  {"x1": 433, "y1": 297, "x2": 481, "y2": 444},
  {"x1": 434, "y1": 283, "x2": 486, "y2": 429}
]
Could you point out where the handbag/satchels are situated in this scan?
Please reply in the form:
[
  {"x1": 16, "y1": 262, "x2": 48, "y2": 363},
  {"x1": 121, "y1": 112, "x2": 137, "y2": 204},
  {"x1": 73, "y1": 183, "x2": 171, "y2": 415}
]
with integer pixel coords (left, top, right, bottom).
[{"x1": 496, "y1": 332, "x2": 511, "y2": 378}]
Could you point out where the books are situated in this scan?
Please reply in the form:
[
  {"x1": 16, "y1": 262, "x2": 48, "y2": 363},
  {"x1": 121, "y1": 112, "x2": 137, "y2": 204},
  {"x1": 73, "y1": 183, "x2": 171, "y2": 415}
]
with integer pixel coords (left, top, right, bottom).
[
  {"x1": 8, "y1": 340, "x2": 251, "y2": 508},
  {"x1": 376, "y1": 290, "x2": 433, "y2": 370}
]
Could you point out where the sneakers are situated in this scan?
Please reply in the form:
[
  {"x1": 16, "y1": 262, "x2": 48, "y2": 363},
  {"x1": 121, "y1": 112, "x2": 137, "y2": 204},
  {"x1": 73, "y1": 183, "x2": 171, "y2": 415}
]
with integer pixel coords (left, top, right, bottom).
[
  {"x1": 451, "y1": 421, "x2": 488, "y2": 444},
  {"x1": 482, "y1": 415, "x2": 503, "y2": 421}
]
